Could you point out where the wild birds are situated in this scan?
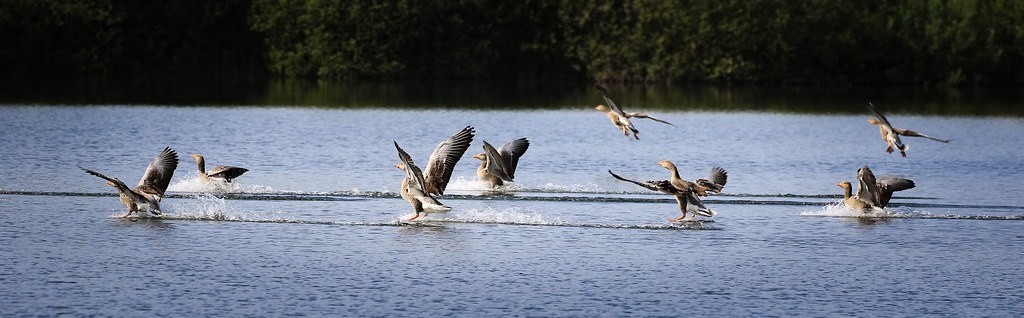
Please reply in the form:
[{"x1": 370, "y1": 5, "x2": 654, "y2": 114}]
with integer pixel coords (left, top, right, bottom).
[
  {"x1": 865, "y1": 103, "x2": 951, "y2": 158},
  {"x1": 594, "y1": 85, "x2": 677, "y2": 140},
  {"x1": 192, "y1": 154, "x2": 249, "y2": 183},
  {"x1": 392, "y1": 125, "x2": 476, "y2": 222},
  {"x1": 607, "y1": 160, "x2": 727, "y2": 222},
  {"x1": 836, "y1": 166, "x2": 916, "y2": 213},
  {"x1": 473, "y1": 138, "x2": 530, "y2": 188},
  {"x1": 78, "y1": 147, "x2": 179, "y2": 219}
]
